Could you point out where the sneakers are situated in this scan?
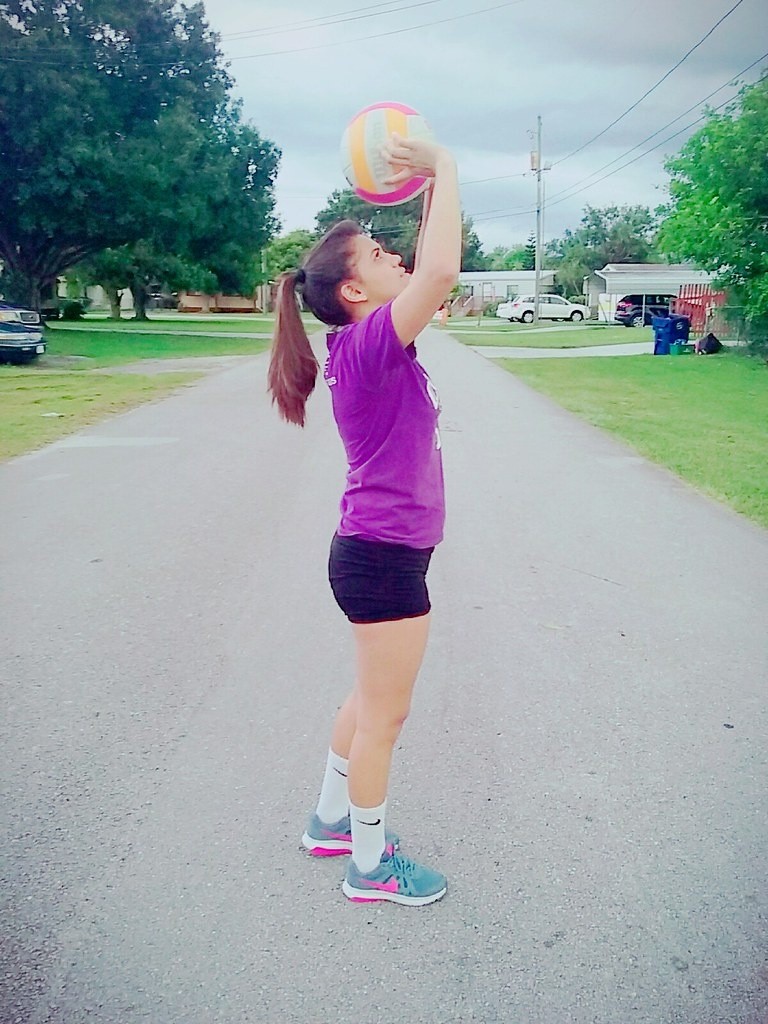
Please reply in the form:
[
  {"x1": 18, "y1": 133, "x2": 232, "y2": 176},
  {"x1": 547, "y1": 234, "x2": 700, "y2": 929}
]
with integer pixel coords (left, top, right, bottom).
[
  {"x1": 302, "y1": 814, "x2": 399, "y2": 857},
  {"x1": 342, "y1": 844, "x2": 448, "y2": 907}
]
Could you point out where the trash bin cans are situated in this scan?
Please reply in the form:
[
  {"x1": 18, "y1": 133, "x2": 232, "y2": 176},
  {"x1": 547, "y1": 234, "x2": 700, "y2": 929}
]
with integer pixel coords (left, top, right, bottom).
[{"x1": 652, "y1": 314, "x2": 691, "y2": 355}]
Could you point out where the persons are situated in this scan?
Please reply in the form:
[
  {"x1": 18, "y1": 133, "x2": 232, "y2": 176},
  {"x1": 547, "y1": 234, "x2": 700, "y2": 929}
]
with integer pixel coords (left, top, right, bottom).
[{"x1": 267, "y1": 131, "x2": 462, "y2": 906}]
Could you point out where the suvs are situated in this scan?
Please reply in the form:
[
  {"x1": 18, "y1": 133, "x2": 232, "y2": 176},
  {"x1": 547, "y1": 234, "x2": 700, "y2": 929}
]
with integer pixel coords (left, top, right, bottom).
[{"x1": 614, "y1": 294, "x2": 702, "y2": 328}]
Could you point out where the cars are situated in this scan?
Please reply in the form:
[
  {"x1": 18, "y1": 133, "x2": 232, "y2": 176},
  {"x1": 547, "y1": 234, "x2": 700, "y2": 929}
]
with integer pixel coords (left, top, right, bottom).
[
  {"x1": 0, "y1": 294, "x2": 47, "y2": 364},
  {"x1": 497, "y1": 294, "x2": 591, "y2": 324}
]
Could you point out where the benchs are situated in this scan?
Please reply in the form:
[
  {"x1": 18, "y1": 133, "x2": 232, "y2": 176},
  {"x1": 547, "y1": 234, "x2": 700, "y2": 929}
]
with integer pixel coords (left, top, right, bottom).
[{"x1": 40, "y1": 306, "x2": 60, "y2": 319}]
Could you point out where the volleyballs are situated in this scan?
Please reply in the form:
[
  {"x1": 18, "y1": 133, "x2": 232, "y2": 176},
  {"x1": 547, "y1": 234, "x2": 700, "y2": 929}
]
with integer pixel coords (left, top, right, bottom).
[{"x1": 337, "y1": 101, "x2": 439, "y2": 208}]
